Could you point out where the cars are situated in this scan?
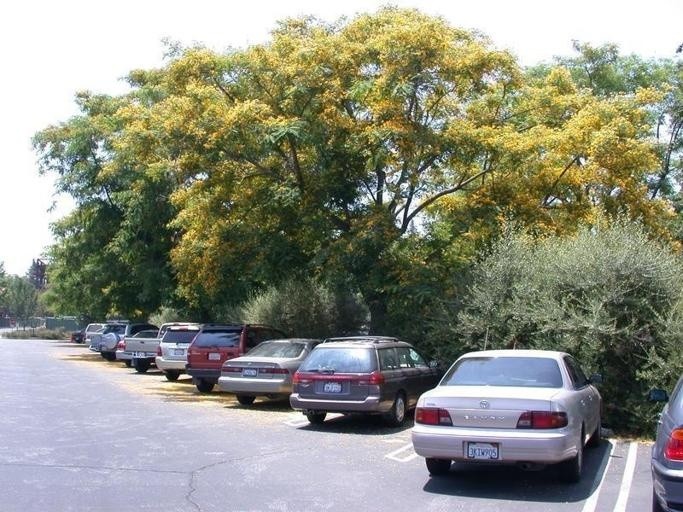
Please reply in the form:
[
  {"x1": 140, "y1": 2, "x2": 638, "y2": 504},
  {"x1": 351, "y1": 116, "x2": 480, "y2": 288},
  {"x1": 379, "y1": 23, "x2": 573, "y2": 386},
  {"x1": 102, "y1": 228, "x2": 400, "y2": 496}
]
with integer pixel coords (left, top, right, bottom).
[
  {"x1": 214, "y1": 339, "x2": 307, "y2": 405},
  {"x1": 158, "y1": 324, "x2": 197, "y2": 378},
  {"x1": 410, "y1": 339, "x2": 609, "y2": 479}
]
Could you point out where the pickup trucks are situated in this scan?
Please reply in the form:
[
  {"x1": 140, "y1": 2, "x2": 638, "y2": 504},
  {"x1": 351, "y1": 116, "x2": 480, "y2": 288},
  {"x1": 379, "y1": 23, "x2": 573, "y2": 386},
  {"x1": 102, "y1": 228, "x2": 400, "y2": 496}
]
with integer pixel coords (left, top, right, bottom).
[{"x1": 128, "y1": 327, "x2": 168, "y2": 373}]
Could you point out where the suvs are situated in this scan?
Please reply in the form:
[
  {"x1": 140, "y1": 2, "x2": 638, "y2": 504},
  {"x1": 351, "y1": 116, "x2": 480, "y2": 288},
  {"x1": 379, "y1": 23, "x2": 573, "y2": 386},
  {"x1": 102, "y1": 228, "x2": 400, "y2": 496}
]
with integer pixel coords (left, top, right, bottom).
[
  {"x1": 648, "y1": 369, "x2": 682, "y2": 506},
  {"x1": 189, "y1": 323, "x2": 287, "y2": 388},
  {"x1": 77, "y1": 320, "x2": 153, "y2": 364},
  {"x1": 292, "y1": 336, "x2": 434, "y2": 423}
]
